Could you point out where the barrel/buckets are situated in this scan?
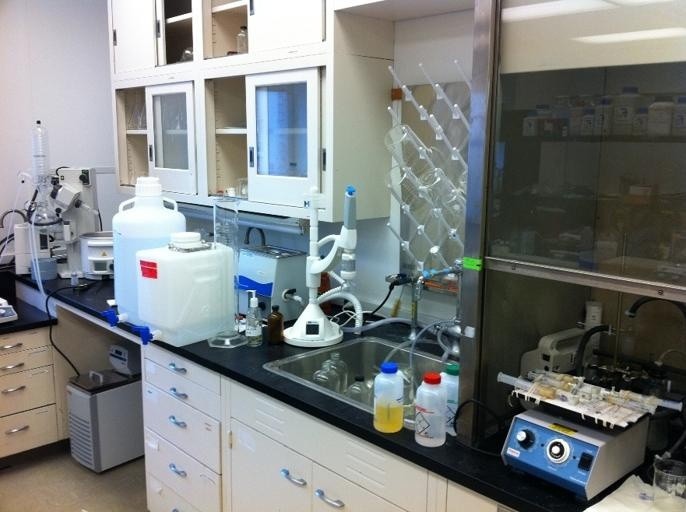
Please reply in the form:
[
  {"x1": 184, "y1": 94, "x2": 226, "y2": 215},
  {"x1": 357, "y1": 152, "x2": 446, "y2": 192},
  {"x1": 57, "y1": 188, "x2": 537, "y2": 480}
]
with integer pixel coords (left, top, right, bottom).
[
  {"x1": 137, "y1": 230, "x2": 237, "y2": 348},
  {"x1": 112, "y1": 177, "x2": 187, "y2": 321}
]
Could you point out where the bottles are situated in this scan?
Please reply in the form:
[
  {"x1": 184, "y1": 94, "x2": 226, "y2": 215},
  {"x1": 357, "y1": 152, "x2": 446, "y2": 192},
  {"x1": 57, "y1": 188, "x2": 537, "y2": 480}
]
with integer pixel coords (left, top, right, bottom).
[
  {"x1": 438, "y1": 361, "x2": 458, "y2": 430},
  {"x1": 313, "y1": 352, "x2": 348, "y2": 397},
  {"x1": 523, "y1": 86, "x2": 686, "y2": 142},
  {"x1": 373, "y1": 362, "x2": 404, "y2": 434},
  {"x1": 237, "y1": 25, "x2": 248, "y2": 54},
  {"x1": 267, "y1": 304, "x2": 284, "y2": 345},
  {"x1": 388, "y1": 361, "x2": 417, "y2": 405},
  {"x1": 414, "y1": 371, "x2": 447, "y2": 448},
  {"x1": 71, "y1": 270, "x2": 79, "y2": 285},
  {"x1": 348, "y1": 376, "x2": 372, "y2": 407}
]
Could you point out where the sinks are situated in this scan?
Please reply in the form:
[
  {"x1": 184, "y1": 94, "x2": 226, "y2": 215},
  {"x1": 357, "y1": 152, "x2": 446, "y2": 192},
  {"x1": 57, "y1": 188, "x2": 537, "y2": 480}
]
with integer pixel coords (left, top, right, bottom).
[{"x1": 263, "y1": 334, "x2": 463, "y2": 432}]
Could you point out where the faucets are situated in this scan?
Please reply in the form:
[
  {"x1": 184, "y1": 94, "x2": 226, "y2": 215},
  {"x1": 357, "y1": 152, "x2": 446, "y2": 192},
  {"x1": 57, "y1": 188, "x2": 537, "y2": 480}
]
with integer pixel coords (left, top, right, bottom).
[
  {"x1": 101, "y1": 310, "x2": 129, "y2": 327},
  {"x1": 131, "y1": 325, "x2": 164, "y2": 345}
]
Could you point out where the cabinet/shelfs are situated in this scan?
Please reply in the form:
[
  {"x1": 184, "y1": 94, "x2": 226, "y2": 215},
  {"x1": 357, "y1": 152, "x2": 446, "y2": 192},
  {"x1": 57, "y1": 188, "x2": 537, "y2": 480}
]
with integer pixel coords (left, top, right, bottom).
[
  {"x1": 445, "y1": 479, "x2": 516, "y2": 511},
  {"x1": 106, "y1": 0, "x2": 396, "y2": 223},
  {"x1": 0, "y1": 326, "x2": 58, "y2": 460},
  {"x1": 143, "y1": 345, "x2": 226, "y2": 512},
  {"x1": 225, "y1": 378, "x2": 446, "y2": 512}
]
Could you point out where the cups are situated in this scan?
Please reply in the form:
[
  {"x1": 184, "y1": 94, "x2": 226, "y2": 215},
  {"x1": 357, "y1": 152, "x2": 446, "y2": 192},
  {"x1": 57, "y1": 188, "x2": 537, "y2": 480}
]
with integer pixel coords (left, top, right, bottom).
[
  {"x1": 207, "y1": 196, "x2": 249, "y2": 350},
  {"x1": 652, "y1": 458, "x2": 686, "y2": 512},
  {"x1": 385, "y1": 126, "x2": 462, "y2": 215}
]
